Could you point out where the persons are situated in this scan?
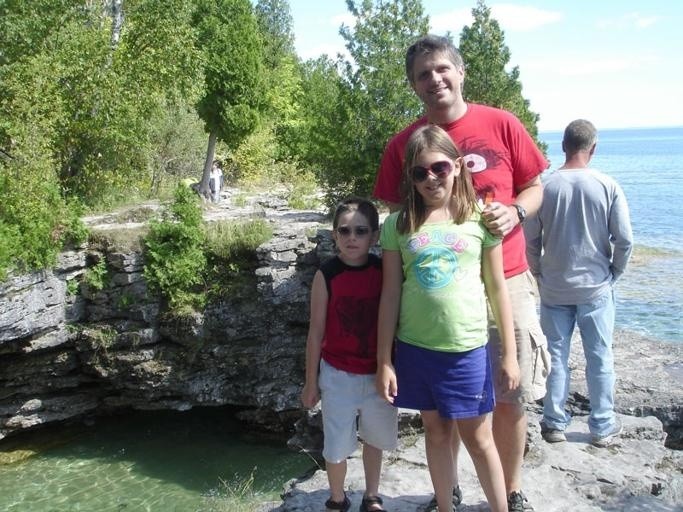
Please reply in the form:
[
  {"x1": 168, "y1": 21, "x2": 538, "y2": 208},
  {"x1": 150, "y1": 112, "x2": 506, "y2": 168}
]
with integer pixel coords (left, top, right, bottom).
[
  {"x1": 208, "y1": 160, "x2": 225, "y2": 204},
  {"x1": 522, "y1": 117, "x2": 635, "y2": 449},
  {"x1": 371, "y1": 35, "x2": 552, "y2": 512},
  {"x1": 374, "y1": 125, "x2": 525, "y2": 512},
  {"x1": 300, "y1": 198, "x2": 398, "y2": 512}
]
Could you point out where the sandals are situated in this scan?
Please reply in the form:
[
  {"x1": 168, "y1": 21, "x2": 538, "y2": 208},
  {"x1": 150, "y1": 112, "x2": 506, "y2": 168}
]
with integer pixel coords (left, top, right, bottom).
[{"x1": 319, "y1": 483, "x2": 538, "y2": 512}]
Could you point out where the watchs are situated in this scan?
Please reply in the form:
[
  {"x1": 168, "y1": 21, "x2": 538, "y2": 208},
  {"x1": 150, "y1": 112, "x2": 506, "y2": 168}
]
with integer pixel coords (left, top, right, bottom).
[{"x1": 511, "y1": 203, "x2": 526, "y2": 224}]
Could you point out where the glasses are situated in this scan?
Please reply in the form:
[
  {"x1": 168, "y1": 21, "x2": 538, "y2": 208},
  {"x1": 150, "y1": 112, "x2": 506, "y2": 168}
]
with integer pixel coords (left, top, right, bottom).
[
  {"x1": 332, "y1": 226, "x2": 370, "y2": 236},
  {"x1": 407, "y1": 161, "x2": 452, "y2": 185}
]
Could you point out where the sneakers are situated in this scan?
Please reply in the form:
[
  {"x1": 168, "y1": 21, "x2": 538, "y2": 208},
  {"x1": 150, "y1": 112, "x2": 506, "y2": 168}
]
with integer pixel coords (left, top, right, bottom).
[
  {"x1": 589, "y1": 415, "x2": 623, "y2": 446},
  {"x1": 540, "y1": 413, "x2": 566, "y2": 441}
]
